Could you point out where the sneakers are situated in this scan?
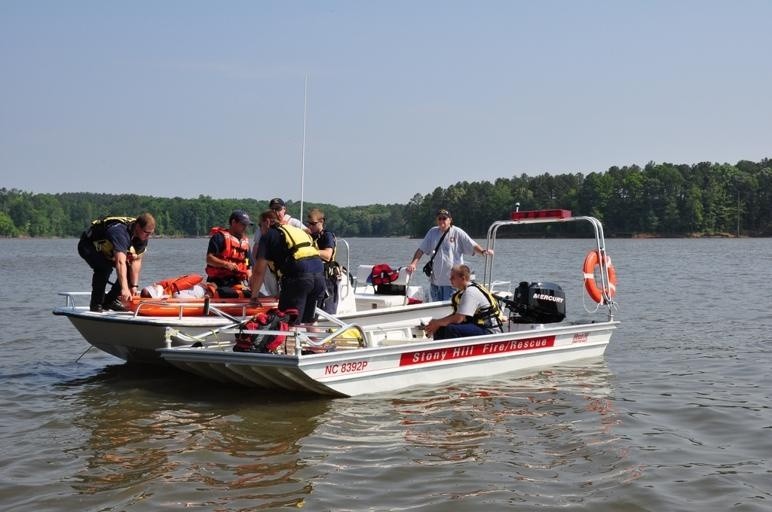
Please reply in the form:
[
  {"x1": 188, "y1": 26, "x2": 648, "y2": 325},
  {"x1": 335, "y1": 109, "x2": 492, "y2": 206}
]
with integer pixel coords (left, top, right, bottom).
[
  {"x1": 104, "y1": 294, "x2": 125, "y2": 312},
  {"x1": 90, "y1": 302, "x2": 104, "y2": 313}
]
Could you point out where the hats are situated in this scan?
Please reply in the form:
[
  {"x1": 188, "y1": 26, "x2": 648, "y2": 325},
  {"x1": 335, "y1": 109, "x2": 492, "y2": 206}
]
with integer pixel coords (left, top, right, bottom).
[
  {"x1": 268, "y1": 197, "x2": 286, "y2": 208},
  {"x1": 230, "y1": 208, "x2": 254, "y2": 227},
  {"x1": 435, "y1": 206, "x2": 452, "y2": 218}
]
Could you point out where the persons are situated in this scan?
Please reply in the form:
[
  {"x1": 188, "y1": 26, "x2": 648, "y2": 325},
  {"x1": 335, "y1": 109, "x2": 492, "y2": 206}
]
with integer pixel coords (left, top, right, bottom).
[
  {"x1": 407, "y1": 208, "x2": 495, "y2": 301},
  {"x1": 252, "y1": 199, "x2": 313, "y2": 296},
  {"x1": 79, "y1": 211, "x2": 156, "y2": 312},
  {"x1": 250, "y1": 211, "x2": 325, "y2": 324},
  {"x1": 303, "y1": 208, "x2": 340, "y2": 316},
  {"x1": 206, "y1": 208, "x2": 254, "y2": 292},
  {"x1": 425, "y1": 264, "x2": 503, "y2": 340}
]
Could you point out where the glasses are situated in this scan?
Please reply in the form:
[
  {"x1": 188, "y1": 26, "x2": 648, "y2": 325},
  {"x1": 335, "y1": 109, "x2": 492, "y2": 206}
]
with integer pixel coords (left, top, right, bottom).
[{"x1": 306, "y1": 220, "x2": 320, "y2": 226}]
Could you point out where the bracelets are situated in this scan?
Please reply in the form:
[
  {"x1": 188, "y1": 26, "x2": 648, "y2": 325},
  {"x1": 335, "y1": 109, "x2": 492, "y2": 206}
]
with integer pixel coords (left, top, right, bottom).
[{"x1": 130, "y1": 283, "x2": 140, "y2": 288}]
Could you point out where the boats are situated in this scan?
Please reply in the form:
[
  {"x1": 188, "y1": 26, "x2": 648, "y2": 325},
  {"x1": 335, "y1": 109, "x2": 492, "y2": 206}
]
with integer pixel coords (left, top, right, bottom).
[
  {"x1": 155, "y1": 194, "x2": 624, "y2": 406},
  {"x1": 53, "y1": 235, "x2": 511, "y2": 364}
]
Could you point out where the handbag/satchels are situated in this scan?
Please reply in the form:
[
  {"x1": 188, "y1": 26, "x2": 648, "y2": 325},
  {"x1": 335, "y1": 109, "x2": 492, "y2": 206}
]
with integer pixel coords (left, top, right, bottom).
[{"x1": 422, "y1": 259, "x2": 432, "y2": 276}]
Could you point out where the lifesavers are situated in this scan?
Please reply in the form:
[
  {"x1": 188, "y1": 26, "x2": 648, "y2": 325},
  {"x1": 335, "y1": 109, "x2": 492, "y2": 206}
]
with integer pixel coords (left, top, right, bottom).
[{"x1": 583, "y1": 249, "x2": 617, "y2": 304}]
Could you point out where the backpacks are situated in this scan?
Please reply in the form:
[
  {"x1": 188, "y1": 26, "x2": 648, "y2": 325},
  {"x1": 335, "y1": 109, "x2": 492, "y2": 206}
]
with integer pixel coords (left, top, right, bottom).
[{"x1": 232, "y1": 304, "x2": 301, "y2": 352}]
